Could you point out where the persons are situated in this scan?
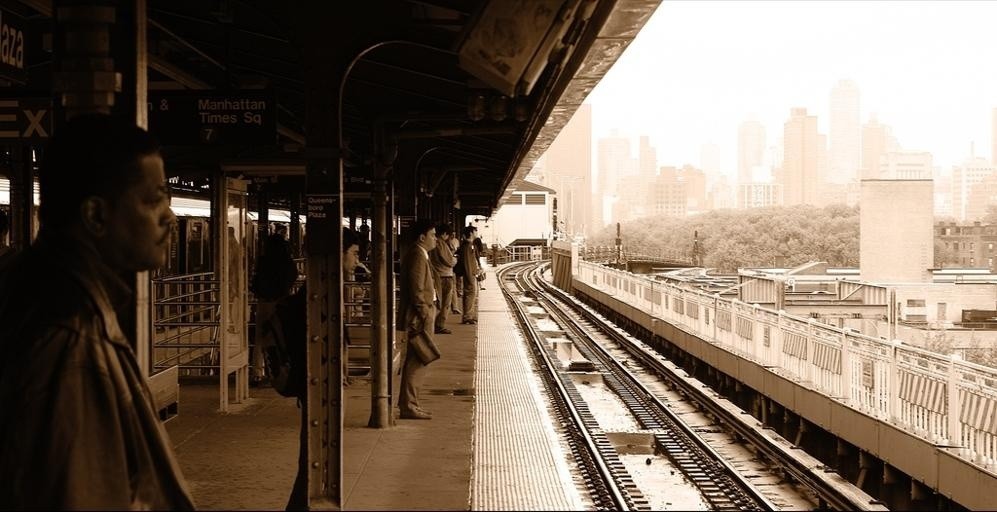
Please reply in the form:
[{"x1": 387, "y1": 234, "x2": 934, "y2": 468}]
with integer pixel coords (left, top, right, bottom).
[
  {"x1": 0, "y1": 113, "x2": 198, "y2": 511},
  {"x1": 286, "y1": 226, "x2": 360, "y2": 511},
  {"x1": 0, "y1": 209, "x2": 13, "y2": 261},
  {"x1": 245, "y1": 218, "x2": 482, "y2": 421}
]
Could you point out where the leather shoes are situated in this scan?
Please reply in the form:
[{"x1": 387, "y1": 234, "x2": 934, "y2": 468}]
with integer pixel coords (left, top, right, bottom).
[{"x1": 400, "y1": 409, "x2": 432, "y2": 419}]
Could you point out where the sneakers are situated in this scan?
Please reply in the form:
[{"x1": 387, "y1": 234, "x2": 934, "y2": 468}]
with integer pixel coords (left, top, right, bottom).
[
  {"x1": 435, "y1": 329, "x2": 451, "y2": 334},
  {"x1": 464, "y1": 318, "x2": 476, "y2": 325}
]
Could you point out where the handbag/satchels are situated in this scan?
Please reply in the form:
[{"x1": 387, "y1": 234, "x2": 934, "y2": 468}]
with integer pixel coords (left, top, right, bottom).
[{"x1": 410, "y1": 330, "x2": 440, "y2": 367}]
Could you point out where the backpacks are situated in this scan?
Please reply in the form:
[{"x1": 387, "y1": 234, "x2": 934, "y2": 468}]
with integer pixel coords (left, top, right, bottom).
[{"x1": 256, "y1": 299, "x2": 306, "y2": 396}]
